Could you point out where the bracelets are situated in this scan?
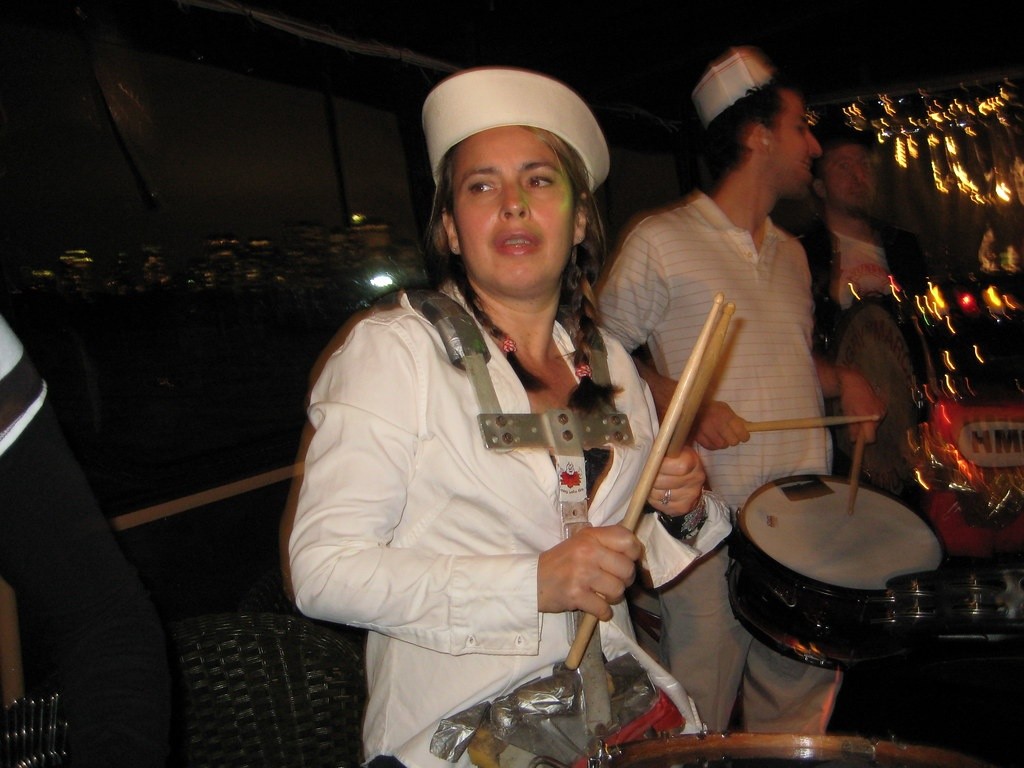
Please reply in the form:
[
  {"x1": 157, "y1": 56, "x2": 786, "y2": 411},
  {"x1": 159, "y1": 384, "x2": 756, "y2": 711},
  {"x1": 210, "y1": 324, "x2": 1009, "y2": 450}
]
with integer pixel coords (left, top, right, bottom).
[{"x1": 657, "y1": 487, "x2": 706, "y2": 543}]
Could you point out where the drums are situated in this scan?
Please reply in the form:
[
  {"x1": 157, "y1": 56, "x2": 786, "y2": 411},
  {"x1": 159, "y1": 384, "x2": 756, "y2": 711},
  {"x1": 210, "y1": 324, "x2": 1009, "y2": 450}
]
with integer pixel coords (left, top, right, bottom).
[
  {"x1": 725, "y1": 471, "x2": 950, "y2": 672},
  {"x1": 588, "y1": 730, "x2": 1001, "y2": 768}
]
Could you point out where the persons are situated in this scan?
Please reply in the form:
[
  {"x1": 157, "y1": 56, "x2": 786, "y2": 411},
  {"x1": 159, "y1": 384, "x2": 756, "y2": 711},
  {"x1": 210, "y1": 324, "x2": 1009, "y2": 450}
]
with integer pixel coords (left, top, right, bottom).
[
  {"x1": 597, "y1": 41, "x2": 883, "y2": 737},
  {"x1": 1, "y1": 308, "x2": 175, "y2": 768},
  {"x1": 805, "y1": 136, "x2": 923, "y2": 493},
  {"x1": 284, "y1": 65, "x2": 733, "y2": 768}
]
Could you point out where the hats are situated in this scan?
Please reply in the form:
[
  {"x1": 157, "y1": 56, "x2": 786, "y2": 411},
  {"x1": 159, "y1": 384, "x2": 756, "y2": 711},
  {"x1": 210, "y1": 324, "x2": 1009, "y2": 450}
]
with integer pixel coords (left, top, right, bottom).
[
  {"x1": 692, "y1": 47, "x2": 778, "y2": 130},
  {"x1": 421, "y1": 67, "x2": 610, "y2": 195}
]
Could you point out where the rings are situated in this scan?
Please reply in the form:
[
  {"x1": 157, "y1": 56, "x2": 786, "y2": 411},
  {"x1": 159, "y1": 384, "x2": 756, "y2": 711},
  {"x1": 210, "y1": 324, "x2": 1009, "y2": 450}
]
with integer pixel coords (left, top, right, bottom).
[{"x1": 661, "y1": 489, "x2": 671, "y2": 505}]
